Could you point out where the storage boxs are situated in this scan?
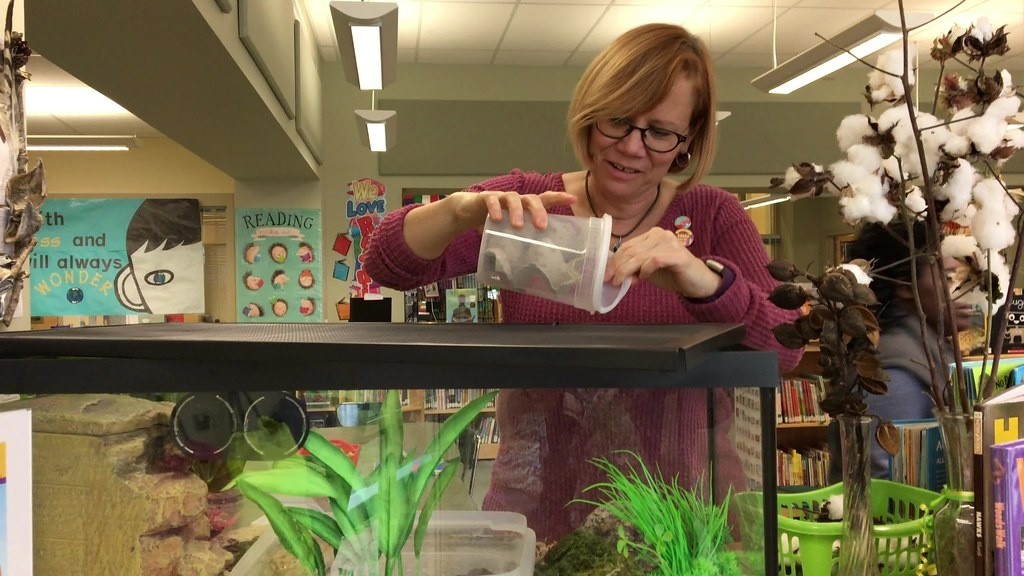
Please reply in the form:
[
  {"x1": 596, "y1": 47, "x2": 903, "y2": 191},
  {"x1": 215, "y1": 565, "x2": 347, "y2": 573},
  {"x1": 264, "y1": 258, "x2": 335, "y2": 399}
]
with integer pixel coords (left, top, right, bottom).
[{"x1": 733, "y1": 472, "x2": 952, "y2": 576}]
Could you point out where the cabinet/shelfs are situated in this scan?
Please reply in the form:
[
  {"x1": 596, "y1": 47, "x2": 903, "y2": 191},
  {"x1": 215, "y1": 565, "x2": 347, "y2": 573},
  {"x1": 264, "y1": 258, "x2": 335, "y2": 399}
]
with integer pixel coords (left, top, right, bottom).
[{"x1": 772, "y1": 341, "x2": 1024, "y2": 475}]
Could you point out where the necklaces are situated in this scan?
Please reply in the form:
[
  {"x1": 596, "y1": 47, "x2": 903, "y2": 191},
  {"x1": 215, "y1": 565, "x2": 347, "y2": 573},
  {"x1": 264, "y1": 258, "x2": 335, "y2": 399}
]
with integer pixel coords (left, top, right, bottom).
[{"x1": 584, "y1": 169, "x2": 661, "y2": 253}]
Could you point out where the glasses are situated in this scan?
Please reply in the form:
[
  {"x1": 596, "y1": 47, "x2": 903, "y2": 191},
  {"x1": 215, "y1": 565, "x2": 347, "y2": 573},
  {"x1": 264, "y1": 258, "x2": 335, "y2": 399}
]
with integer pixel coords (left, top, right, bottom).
[{"x1": 594, "y1": 113, "x2": 688, "y2": 154}]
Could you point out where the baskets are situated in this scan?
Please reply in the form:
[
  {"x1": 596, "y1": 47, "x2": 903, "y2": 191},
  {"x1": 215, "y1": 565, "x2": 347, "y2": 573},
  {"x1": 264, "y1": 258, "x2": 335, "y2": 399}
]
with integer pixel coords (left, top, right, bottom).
[{"x1": 730, "y1": 472, "x2": 948, "y2": 576}]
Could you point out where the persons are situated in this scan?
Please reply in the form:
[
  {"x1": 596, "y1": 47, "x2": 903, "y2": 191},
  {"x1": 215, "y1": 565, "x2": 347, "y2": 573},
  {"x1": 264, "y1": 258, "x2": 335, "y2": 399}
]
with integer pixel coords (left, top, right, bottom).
[
  {"x1": 826, "y1": 214, "x2": 978, "y2": 486},
  {"x1": 365, "y1": 22, "x2": 808, "y2": 573}
]
